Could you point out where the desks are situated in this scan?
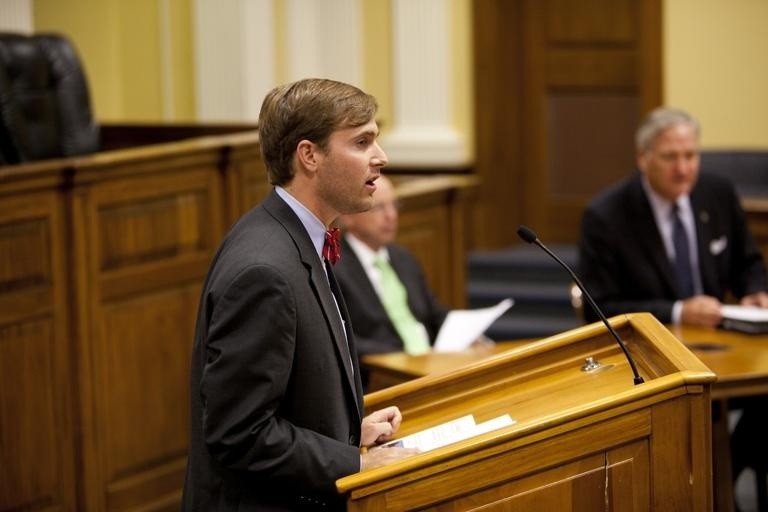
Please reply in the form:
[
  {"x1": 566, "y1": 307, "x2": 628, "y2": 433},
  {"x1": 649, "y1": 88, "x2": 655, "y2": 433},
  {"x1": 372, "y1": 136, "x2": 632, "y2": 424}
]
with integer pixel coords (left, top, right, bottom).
[{"x1": 361, "y1": 322, "x2": 768, "y2": 512}]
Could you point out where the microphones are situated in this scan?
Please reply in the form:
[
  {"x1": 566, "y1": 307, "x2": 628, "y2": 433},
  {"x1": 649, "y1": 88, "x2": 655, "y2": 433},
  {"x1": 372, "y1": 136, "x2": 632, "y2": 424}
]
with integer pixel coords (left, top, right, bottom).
[{"x1": 516, "y1": 225, "x2": 645, "y2": 385}]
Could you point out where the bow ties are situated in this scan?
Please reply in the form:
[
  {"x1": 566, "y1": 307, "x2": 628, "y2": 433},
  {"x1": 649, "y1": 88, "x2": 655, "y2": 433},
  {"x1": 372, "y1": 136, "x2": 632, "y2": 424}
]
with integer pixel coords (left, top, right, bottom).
[{"x1": 322, "y1": 228, "x2": 343, "y2": 267}]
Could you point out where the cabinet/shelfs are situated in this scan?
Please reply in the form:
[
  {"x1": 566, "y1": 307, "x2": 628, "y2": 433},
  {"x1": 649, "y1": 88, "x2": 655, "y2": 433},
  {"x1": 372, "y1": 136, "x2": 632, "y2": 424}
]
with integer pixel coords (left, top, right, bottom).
[
  {"x1": 389, "y1": 168, "x2": 480, "y2": 311},
  {"x1": 2, "y1": 124, "x2": 270, "y2": 509}
]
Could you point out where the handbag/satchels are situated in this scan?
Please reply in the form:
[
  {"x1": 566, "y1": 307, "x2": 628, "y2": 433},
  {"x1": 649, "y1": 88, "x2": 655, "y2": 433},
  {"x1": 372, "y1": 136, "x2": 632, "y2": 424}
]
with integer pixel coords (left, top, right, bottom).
[{"x1": 1, "y1": 33, "x2": 102, "y2": 161}]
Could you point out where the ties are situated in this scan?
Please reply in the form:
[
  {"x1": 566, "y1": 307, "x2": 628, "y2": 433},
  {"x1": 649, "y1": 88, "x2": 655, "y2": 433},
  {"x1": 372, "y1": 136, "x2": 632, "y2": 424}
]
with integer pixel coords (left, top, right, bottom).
[
  {"x1": 374, "y1": 260, "x2": 420, "y2": 353},
  {"x1": 664, "y1": 203, "x2": 694, "y2": 299}
]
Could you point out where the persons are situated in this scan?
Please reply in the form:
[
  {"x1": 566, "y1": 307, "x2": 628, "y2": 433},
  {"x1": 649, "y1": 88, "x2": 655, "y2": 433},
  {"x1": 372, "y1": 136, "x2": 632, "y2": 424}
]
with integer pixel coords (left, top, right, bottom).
[
  {"x1": 177, "y1": 77, "x2": 423, "y2": 511},
  {"x1": 578, "y1": 105, "x2": 768, "y2": 511},
  {"x1": 331, "y1": 174, "x2": 495, "y2": 392}
]
